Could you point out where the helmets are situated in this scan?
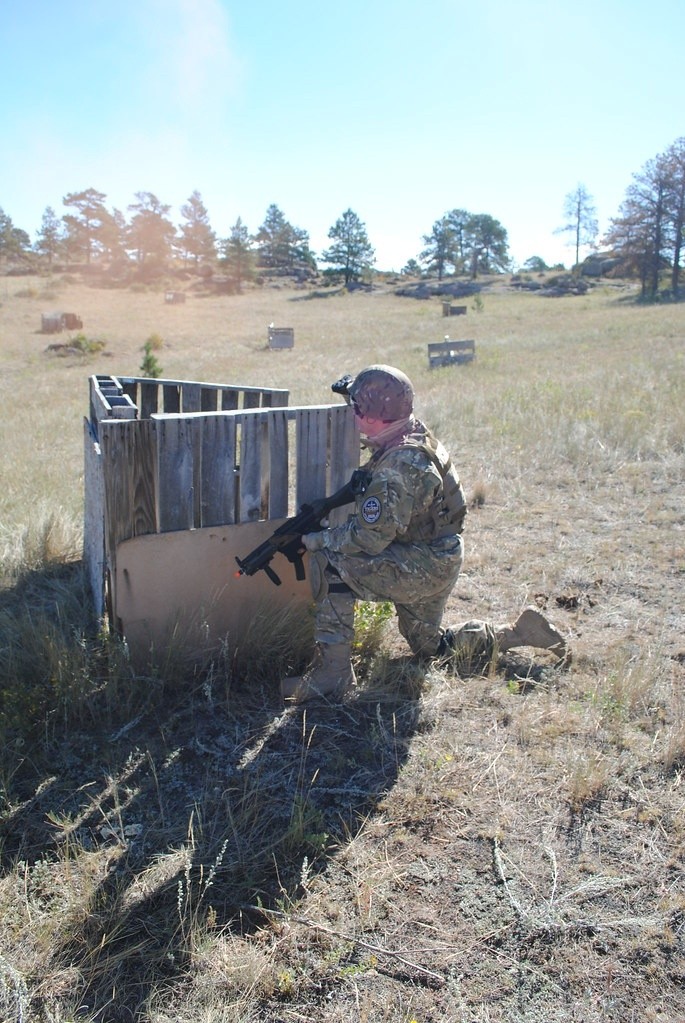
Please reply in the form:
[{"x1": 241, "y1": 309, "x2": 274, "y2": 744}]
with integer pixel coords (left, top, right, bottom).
[{"x1": 346, "y1": 363, "x2": 415, "y2": 420}]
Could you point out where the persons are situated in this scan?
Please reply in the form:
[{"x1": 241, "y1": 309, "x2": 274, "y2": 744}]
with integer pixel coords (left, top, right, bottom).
[{"x1": 280, "y1": 364, "x2": 573, "y2": 697}]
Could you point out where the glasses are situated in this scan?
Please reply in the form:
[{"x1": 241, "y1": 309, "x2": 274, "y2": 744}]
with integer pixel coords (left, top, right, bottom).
[{"x1": 349, "y1": 395, "x2": 364, "y2": 419}]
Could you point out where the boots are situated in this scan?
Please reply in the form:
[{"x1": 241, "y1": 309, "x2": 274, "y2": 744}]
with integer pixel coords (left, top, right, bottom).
[
  {"x1": 281, "y1": 641, "x2": 358, "y2": 701},
  {"x1": 495, "y1": 604, "x2": 572, "y2": 662}
]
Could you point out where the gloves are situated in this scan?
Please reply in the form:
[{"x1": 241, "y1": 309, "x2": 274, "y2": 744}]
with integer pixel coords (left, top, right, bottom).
[{"x1": 302, "y1": 529, "x2": 330, "y2": 552}]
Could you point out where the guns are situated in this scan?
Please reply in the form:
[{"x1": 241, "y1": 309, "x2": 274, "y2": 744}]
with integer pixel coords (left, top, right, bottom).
[{"x1": 233, "y1": 470, "x2": 367, "y2": 585}]
